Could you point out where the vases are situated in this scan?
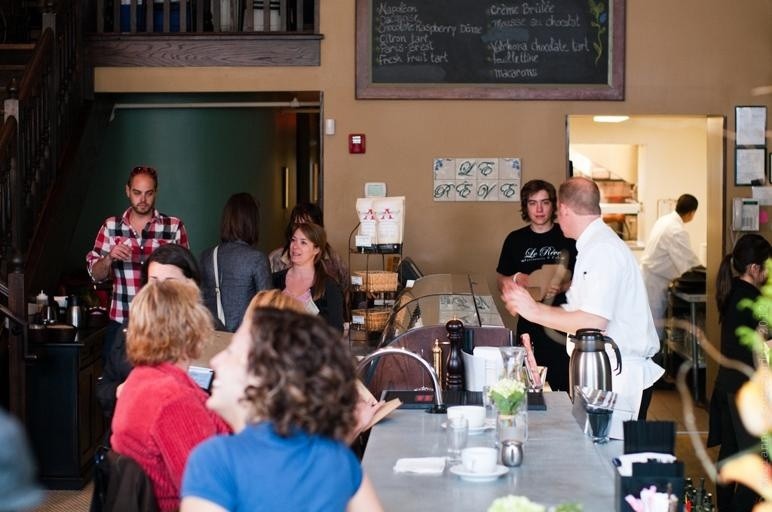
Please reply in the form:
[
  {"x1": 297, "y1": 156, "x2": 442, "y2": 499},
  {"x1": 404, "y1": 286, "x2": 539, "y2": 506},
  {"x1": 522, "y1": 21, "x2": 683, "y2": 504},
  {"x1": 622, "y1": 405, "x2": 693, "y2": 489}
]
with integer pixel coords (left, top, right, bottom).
[{"x1": 494, "y1": 409, "x2": 530, "y2": 447}]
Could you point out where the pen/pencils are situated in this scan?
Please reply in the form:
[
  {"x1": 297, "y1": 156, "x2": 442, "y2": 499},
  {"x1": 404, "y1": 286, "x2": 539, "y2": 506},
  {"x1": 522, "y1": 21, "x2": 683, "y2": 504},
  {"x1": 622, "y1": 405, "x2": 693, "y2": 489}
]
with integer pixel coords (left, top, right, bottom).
[{"x1": 625, "y1": 477, "x2": 715, "y2": 512}]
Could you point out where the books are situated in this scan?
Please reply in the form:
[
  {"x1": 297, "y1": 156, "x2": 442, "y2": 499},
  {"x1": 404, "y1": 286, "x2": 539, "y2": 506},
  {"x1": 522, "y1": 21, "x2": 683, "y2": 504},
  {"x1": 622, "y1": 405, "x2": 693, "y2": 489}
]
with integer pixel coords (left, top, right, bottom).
[{"x1": 353, "y1": 377, "x2": 403, "y2": 432}]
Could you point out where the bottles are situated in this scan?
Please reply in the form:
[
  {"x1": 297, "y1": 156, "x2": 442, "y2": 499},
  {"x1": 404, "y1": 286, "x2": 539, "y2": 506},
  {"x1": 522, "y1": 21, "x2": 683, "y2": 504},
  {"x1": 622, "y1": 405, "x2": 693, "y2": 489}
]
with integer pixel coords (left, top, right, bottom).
[
  {"x1": 42, "y1": 301, "x2": 62, "y2": 321},
  {"x1": 432, "y1": 339, "x2": 442, "y2": 388},
  {"x1": 36, "y1": 289, "x2": 48, "y2": 314},
  {"x1": 445, "y1": 315, "x2": 466, "y2": 389},
  {"x1": 377, "y1": 219, "x2": 400, "y2": 244},
  {"x1": 359, "y1": 219, "x2": 377, "y2": 244},
  {"x1": 501, "y1": 440, "x2": 524, "y2": 468}
]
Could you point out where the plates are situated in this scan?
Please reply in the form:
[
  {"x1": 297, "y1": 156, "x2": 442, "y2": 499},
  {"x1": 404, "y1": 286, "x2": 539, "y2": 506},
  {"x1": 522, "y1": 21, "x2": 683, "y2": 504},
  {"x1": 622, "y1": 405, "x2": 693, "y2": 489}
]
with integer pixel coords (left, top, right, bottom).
[
  {"x1": 441, "y1": 420, "x2": 490, "y2": 436},
  {"x1": 450, "y1": 464, "x2": 510, "y2": 481}
]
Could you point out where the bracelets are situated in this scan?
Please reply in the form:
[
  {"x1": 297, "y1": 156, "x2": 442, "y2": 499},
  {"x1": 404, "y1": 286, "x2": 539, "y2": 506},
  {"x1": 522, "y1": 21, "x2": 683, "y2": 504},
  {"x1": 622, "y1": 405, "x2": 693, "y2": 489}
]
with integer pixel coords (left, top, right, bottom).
[{"x1": 513, "y1": 269, "x2": 523, "y2": 285}]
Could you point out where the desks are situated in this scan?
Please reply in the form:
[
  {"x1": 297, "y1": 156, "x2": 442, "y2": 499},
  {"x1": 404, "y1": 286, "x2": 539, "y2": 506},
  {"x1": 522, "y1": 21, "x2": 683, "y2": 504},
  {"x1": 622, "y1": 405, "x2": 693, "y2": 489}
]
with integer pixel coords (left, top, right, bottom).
[{"x1": 360, "y1": 386, "x2": 614, "y2": 511}]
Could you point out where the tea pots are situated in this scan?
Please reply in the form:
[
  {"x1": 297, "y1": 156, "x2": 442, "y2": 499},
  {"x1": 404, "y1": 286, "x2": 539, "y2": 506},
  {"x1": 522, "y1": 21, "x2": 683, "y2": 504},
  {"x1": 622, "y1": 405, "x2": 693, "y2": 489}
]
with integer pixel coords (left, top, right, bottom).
[{"x1": 65, "y1": 294, "x2": 83, "y2": 341}]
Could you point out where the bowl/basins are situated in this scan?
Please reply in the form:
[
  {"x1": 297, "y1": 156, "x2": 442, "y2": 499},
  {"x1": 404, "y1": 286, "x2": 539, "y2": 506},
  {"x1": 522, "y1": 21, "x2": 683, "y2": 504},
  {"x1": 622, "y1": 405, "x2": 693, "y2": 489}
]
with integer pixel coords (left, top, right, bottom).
[{"x1": 447, "y1": 406, "x2": 486, "y2": 427}]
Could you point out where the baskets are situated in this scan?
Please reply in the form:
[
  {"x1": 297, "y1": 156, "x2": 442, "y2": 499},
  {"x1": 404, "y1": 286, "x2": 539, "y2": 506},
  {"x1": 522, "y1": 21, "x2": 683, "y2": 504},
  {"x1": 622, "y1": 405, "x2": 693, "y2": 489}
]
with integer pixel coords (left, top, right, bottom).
[
  {"x1": 352, "y1": 270, "x2": 399, "y2": 293},
  {"x1": 352, "y1": 306, "x2": 391, "y2": 332}
]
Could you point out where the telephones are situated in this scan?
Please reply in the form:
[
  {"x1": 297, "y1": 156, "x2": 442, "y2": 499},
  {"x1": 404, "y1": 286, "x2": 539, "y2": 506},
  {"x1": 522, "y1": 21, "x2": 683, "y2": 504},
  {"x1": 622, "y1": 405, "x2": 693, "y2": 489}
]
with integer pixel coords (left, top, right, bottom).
[{"x1": 732, "y1": 197, "x2": 760, "y2": 231}]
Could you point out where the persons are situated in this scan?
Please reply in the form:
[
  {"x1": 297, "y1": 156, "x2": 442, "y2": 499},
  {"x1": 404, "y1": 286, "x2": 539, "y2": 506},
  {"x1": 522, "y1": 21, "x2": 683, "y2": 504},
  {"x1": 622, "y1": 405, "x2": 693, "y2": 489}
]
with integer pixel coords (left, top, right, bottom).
[
  {"x1": 196, "y1": 193, "x2": 272, "y2": 336},
  {"x1": 271, "y1": 218, "x2": 345, "y2": 334},
  {"x1": 495, "y1": 178, "x2": 578, "y2": 391},
  {"x1": 83, "y1": 167, "x2": 189, "y2": 379},
  {"x1": 110, "y1": 278, "x2": 385, "y2": 511},
  {"x1": 178, "y1": 307, "x2": 385, "y2": 512},
  {"x1": 708, "y1": 231, "x2": 772, "y2": 512},
  {"x1": 502, "y1": 178, "x2": 666, "y2": 431},
  {"x1": 268, "y1": 203, "x2": 350, "y2": 302},
  {"x1": 640, "y1": 193, "x2": 702, "y2": 392},
  {"x1": 92, "y1": 243, "x2": 228, "y2": 448}
]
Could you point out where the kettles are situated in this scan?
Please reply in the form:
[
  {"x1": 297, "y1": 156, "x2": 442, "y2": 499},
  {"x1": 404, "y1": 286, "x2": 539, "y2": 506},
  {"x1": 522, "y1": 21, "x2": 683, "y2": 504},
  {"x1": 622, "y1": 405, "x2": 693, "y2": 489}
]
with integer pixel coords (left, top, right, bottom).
[{"x1": 567, "y1": 328, "x2": 623, "y2": 405}]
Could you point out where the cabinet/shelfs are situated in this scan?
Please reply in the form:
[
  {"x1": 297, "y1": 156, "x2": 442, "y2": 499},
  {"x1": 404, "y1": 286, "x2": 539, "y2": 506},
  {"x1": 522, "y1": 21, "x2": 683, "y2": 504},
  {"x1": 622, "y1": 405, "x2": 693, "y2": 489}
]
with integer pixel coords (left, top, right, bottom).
[
  {"x1": 26, "y1": 328, "x2": 114, "y2": 491},
  {"x1": 347, "y1": 222, "x2": 403, "y2": 362}
]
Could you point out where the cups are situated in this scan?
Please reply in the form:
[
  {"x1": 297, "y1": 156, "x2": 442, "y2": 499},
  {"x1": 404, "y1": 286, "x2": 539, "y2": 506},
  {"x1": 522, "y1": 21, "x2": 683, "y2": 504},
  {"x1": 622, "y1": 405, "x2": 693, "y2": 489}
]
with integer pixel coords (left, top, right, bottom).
[
  {"x1": 53, "y1": 296, "x2": 68, "y2": 316},
  {"x1": 446, "y1": 418, "x2": 469, "y2": 464},
  {"x1": 122, "y1": 248, "x2": 133, "y2": 263},
  {"x1": 461, "y1": 446, "x2": 496, "y2": 474},
  {"x1": 586, "y1": 410, "x2": 613, "y2": 446}
]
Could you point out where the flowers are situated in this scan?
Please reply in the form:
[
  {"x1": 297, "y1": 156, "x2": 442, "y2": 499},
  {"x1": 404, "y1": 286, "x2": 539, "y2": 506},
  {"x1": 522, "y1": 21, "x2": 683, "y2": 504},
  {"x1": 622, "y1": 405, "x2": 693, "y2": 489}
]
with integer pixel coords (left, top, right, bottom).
[{"x1": 486, "y1": 375, "x2": 528, "y2": 430}]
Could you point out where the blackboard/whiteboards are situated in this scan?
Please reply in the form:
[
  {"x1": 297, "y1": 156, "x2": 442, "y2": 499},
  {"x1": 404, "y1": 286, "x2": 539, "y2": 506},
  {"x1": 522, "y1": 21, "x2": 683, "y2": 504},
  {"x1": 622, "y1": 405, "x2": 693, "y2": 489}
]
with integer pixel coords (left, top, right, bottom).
[{"x1": 355, "y1": 0, "x2": 625, "y2": 101}]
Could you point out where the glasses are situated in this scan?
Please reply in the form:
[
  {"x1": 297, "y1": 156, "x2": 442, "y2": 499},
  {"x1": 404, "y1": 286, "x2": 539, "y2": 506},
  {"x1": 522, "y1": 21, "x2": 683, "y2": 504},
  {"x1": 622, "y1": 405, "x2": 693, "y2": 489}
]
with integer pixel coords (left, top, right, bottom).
[{"x1": 128, "y1": 166, "x2": 156, "y2": 177}]
